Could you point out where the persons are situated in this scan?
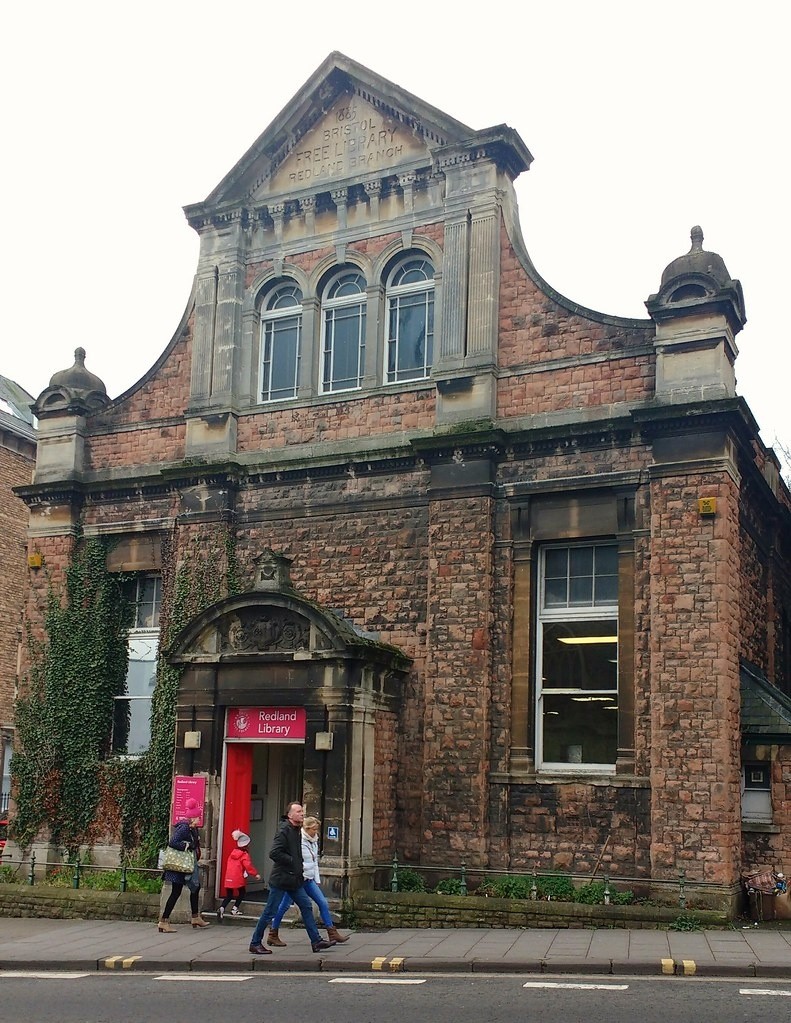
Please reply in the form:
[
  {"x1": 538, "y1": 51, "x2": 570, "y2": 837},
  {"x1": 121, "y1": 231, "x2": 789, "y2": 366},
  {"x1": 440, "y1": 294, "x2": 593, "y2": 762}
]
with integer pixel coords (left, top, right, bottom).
[
  {"x1": 157, "y1": 797, "x2": 209, "y2": 934},
  {"x1": 249, "y1": 801, "x2": 350, "y2": 954},
  {"x1": 216, "y1": 830, "x2": 261, "y2": 920}
]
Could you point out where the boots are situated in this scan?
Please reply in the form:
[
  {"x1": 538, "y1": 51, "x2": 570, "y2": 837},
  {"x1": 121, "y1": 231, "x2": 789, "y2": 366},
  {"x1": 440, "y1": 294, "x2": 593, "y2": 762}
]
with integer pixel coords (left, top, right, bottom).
[
  {"x1": 267, "y1": 928, "x2": 286, "y2": 946},
  {"x1": 327, "y1": 926, "x2": 350, "y2": 943}
]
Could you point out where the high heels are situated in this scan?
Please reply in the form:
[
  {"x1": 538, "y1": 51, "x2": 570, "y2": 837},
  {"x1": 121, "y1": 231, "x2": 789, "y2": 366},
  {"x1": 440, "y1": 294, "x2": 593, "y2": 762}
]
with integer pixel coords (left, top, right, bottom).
[
  {"x1": 158, "y1": 917, "x2": 177, "y2": 932},
  {"x1": 191, "y1": 914, "x2": 210, "y2": 928}
]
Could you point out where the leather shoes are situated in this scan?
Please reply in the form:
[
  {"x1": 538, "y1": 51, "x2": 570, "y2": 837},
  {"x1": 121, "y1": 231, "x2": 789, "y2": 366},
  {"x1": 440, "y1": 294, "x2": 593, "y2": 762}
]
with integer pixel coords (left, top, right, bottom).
[
  {"x1": 312, "y1": 938, "x2": 337, "y2": 953},
  {"x1": 249, "y1": 943, "x2": 272, "y2": 954}
]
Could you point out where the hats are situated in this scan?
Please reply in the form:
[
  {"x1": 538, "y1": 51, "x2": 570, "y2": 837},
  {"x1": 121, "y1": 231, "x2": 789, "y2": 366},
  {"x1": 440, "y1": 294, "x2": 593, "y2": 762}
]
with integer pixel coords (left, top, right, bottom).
[
  {"x1": 232, "y1": 830, "x2": 251, "y2": 847},
  {"x1": 186, "y1": 798, "x2": 201, "y2": 818}
]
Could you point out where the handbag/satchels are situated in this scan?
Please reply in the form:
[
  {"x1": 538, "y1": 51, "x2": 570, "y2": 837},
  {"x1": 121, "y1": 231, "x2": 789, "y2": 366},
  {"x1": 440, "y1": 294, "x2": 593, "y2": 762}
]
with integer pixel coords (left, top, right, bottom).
[{"x1": 163, "y1": 840, "x2": 195, "y2": 874}]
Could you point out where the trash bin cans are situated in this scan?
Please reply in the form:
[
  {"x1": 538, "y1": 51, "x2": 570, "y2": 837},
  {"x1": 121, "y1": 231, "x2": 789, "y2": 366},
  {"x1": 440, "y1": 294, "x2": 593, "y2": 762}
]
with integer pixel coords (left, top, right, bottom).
[{"x1": 746, "y1": 889, "x2": 774, "y2": 921}]
